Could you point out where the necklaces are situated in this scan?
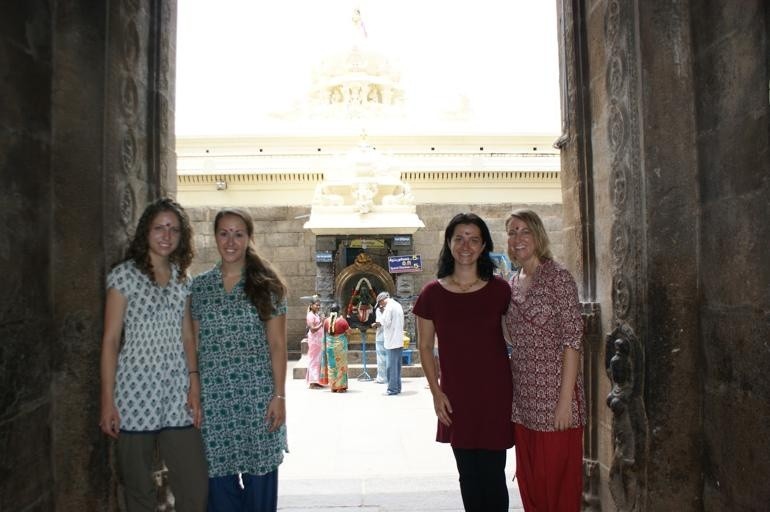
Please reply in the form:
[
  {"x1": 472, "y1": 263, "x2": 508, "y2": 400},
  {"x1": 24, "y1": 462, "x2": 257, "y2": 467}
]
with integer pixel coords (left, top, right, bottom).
[{"x1": 450, "y1": 275, "x2": 481, "y2": 292}]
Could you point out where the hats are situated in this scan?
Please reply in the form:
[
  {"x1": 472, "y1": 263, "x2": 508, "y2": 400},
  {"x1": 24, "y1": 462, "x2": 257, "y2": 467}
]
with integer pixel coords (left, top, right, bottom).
[{"x1": 373, "y1": 291, "x2": 391, "y2": 310}]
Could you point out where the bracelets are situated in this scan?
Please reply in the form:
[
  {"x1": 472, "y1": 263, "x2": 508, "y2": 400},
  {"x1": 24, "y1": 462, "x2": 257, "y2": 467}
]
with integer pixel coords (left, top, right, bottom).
[
  {"x1": 189, "y1": 370, "x2": 200, "y2": 376},
  {"x1": 271, "y1": 395, "x2": 285, "y2": 399}
]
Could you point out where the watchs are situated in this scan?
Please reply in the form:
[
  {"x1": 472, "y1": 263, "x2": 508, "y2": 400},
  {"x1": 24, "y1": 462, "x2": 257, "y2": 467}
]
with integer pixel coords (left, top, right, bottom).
[{"x1": 94, "y1": 197, "x2": 211, "y2": 512}]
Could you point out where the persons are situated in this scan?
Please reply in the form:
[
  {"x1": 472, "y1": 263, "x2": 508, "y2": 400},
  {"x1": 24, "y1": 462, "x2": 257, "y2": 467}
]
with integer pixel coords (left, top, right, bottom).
[
  {"x1": 305, "y1": 297, "x2": 327, "y2": 390},
  {"x1": 319, "y1": 302, "x2": 353, "y2": 392},
  {"x1": 189, "y1": 209, "x2": 291, "y2": 512},
  {"x1": 412, "y1": 213, "x2": 513, "y2": 511},
  {"x1": 371, "y1": 308, "x2": 388, "y2": 384},
  {"x1": 504, "y1": 209, "x2": 588, "y2": 511},
  {"x1": 374, "y1": 291, "x2": 405, "y2": 395}
]
